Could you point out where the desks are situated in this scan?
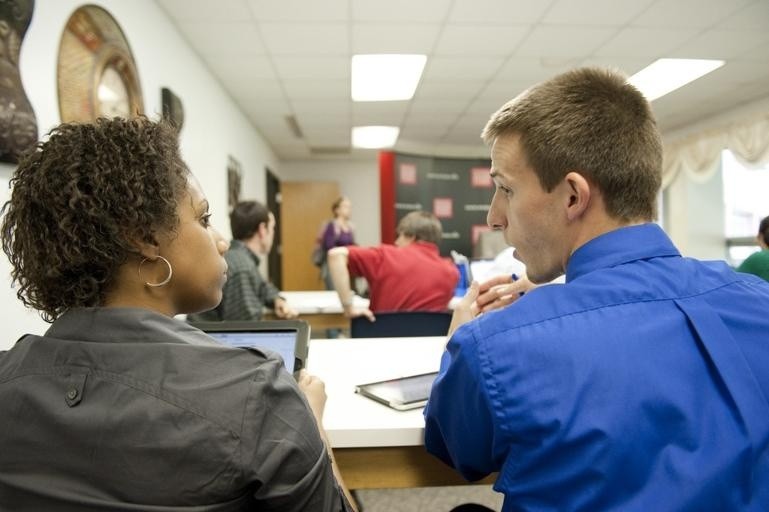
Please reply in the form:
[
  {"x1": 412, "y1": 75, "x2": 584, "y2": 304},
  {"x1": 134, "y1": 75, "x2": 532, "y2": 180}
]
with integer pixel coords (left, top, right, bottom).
[
  {"x1": 308, "y1": 335, "x2": 504, "y2": 511},
  {"x1": 280, "y1": 287, "x2": 353, "y2": 338}
]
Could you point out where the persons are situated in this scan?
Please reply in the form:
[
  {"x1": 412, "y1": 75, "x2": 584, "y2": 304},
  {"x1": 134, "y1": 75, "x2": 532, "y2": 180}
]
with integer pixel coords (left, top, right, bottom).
[
  {"x1": 423, "y1": 65, "x2": 768, "y2": 511},
  {"x1": 183, "y1": 200, "x2": 297, "y2": 326},
  {"x1": 316, "y1": 194, "x2": 361, "y2": 339},
  {"x1": 0, "y1": 112, "x2": 355, "y2": 511},
  {"x1": 325, "y1": 207, "x2": 461, "y2": 325},
  {"x1": 738, "y1": 216, "x2": 769, "y2": 283}
]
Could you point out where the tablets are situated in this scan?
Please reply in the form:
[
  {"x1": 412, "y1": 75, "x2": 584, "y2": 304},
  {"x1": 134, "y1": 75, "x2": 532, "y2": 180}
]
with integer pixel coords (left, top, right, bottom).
[
  {"x1": 355, "y1": 371, "x2": 440, "y2": 411},
  {"x1": 186, "y1": 321, "x2": 310, "y2": 385}
]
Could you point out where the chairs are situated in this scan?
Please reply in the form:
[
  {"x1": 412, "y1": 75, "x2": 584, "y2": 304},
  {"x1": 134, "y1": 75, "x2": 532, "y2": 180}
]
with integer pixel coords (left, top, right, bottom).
[{"x1": 351, "y1": 311, "x2": 452, "y2": 336}]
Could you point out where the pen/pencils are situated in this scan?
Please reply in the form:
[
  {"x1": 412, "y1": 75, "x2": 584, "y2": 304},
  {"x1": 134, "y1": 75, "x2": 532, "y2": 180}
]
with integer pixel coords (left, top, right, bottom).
[{"x1": 512, "y1": 274, "x2": 525, "y2": 296}]
